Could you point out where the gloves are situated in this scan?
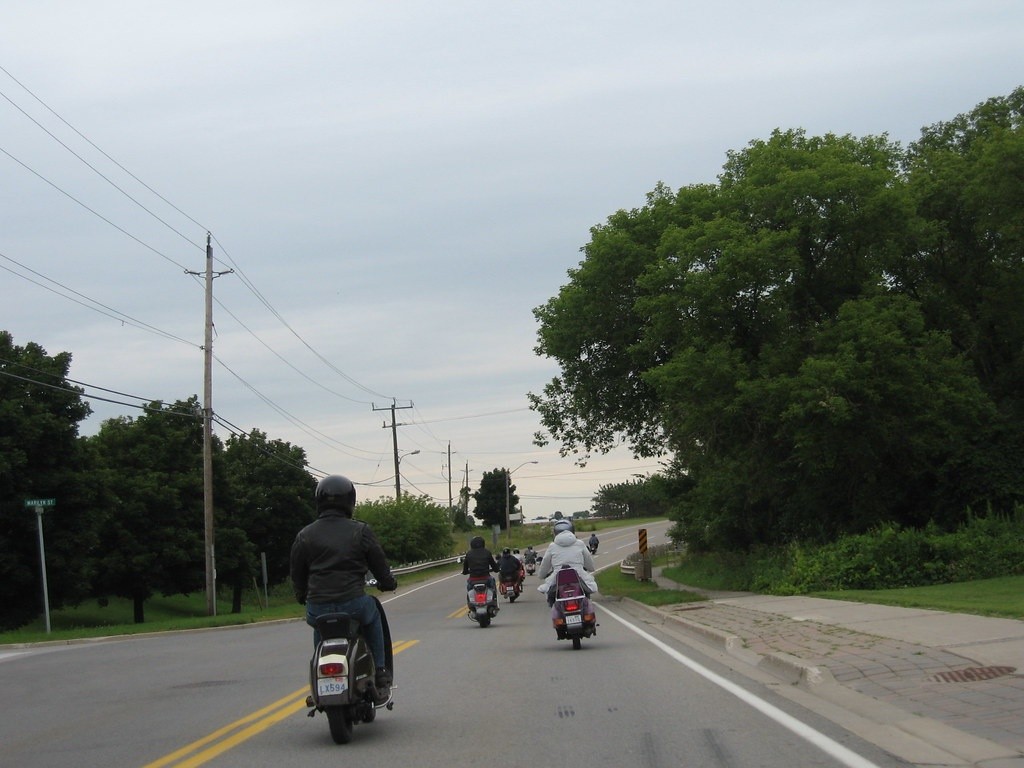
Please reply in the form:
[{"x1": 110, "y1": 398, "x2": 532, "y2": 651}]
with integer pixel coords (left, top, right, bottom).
[
  {"x1": 495, "y1": 568, "x2": 500, "y2": 573},
  {"x1": 378, "y1": 575, "x2": 396, "y2": 591},
  {"x1": 463, "y1": 572, "x2": 468, "y2": 575},
  {"x1": 296, "y1": 592, "x2": 307, "y2": 605}
]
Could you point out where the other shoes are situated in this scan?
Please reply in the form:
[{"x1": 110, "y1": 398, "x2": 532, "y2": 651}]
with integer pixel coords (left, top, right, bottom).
[
  {"x1": 375, "y1": 670, "x2": 392, "y2": 687},
  {"x1": 494, "y1": 606, "x2": 500, "y2": 611}
]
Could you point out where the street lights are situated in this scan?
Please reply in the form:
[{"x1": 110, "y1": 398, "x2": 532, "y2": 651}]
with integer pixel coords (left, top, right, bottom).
[
  {"x1": 505, "y1": 460, "x2": 540, "y2": 539},
  {"x1": 394, "y1": 450, "x2": 420, "y2": 501}
]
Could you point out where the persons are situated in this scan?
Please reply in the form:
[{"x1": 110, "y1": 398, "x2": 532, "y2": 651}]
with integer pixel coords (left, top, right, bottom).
[
  {"x1": 463, "y1": 536, "x2": 499, "y2": 609},
  {"x1": 589, "y1": 534, "x2": 599, "y2": 549},
  {"x1": 537, "y1": 519, "x2": 599, "y2": 619},
  {"x1": 512, "y1": 548, "x2": 526, "y2": 563},
  {"x1": 289, "y1": 475, "x2": 398, "y2": 688},
  {"x1": 497, "y1": 547, "x2": 521, "y2": 585},
  {"x1": 524, "y1": 545, "x2": 537, "y2": 564}
]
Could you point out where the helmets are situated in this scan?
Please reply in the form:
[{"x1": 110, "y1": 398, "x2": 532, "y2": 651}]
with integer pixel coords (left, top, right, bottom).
[
  {"x1": 470, "y1": 536, "x2": 485, "y2": 549},
  {"x1": 496, "y1": 554, "x2": 501, "y2": 560},
  {"x1": 315, "y1": 475, "x2": 356, "y2": 518},
  {"x1": 513, "y1": 548, "x2": 519, "y2": 554},
  {"x1": 592, "y1": 534, "x2": 595, "y2": 537},
  {"x1": 528, "y1": 546, "x2": 532, "y2": 550},
  {"x1": 503, "y1": 547, "x2": 510, "y2": 554},
  {"x1": 554, "y1": 519, "x2": 574, "y2": 537}
]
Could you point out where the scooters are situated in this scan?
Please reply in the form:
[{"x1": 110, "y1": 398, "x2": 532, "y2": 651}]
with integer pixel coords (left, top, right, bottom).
[
  {"x1": 460, "y1": 569, "x2": 502, "y2": 628},
  {"x1": 496, "y1": 567, "x2": 527, "y2": 604},
  {"x1": 303, "y1": 577, "x2": 398, "y2": 746},
  {"x1": 543, "y1": 564, "x2": 601, "y2": 650},
  {"x1": 523, "y1": 552, "x2": 538, "y2": 576},
  {"x1": 589, "y1": 542, "x2": 599, "y2": 555}
]
